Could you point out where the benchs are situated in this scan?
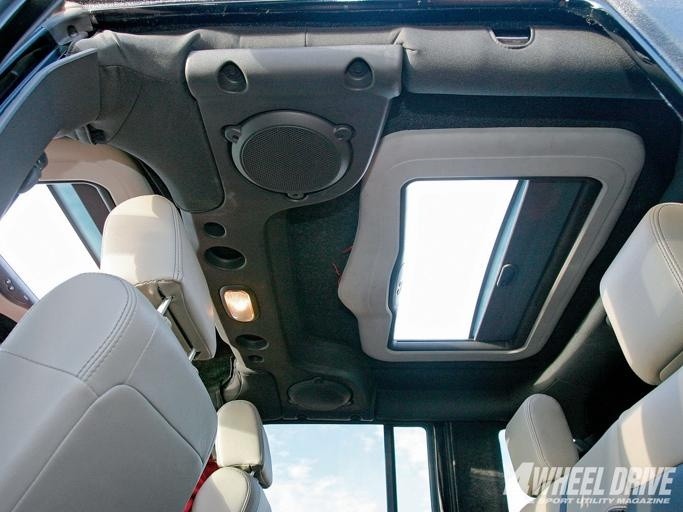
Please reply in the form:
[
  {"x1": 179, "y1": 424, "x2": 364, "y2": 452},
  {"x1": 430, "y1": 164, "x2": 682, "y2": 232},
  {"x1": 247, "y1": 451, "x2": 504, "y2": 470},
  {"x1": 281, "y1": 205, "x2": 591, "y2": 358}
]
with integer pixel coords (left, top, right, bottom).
[{"x1": 498, "y1": 200, "x2": 683, "y2": 510}]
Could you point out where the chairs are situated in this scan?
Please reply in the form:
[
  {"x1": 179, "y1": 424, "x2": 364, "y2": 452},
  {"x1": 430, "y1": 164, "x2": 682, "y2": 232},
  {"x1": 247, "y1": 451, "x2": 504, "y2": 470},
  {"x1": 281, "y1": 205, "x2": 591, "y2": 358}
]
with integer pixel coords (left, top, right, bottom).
[
  {"x1": 191, "y1": 400, "x2": 274, "y2": 512},
  {"x1": 0, "y1": 140, "x2": 216, "y2": 512}
]
